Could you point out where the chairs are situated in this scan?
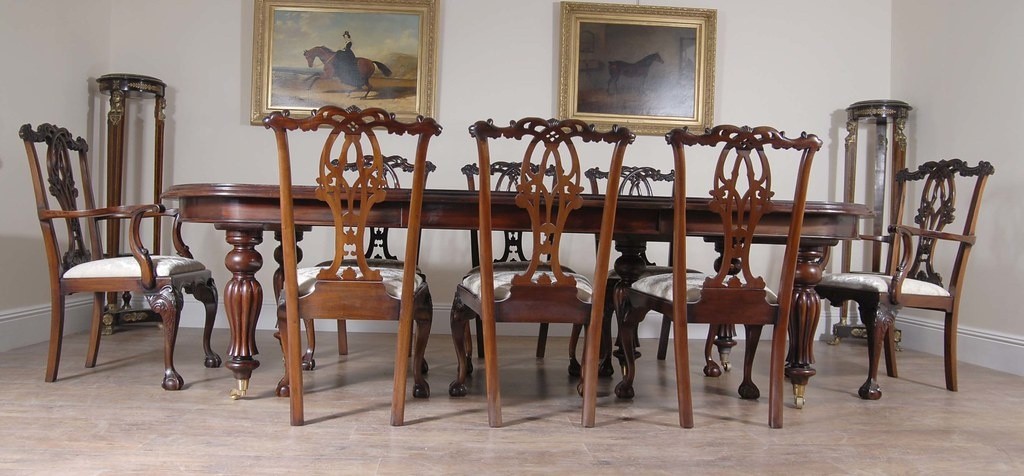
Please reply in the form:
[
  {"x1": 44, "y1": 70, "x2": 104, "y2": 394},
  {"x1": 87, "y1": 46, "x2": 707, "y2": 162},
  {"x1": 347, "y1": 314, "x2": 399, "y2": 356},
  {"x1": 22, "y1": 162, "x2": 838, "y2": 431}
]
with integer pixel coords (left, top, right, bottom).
[
  {"x1": 622, "y1": 124, "x2": 823, "y2": 430},
  {"x1": 583, "y1": 165, "x2": 703, "y2": 359},
  {"x1": 460, "y1": 161, "x2": 565, "y2": 359},
  {"x1": 260, "y1": 104, "x2": 443, "y2": 426},
  {"x1": 301, "y1": 154, "x2": 437, "y2": 369},
  {"x1": 18, "y1": 122, "x2": 222, "y2": 390},
  {"x1": 448, "y1": 117, "x2": 637, "y2": 429},
  {"x1": 816, "y1": 158, "x2": 994, "y2": 393}
]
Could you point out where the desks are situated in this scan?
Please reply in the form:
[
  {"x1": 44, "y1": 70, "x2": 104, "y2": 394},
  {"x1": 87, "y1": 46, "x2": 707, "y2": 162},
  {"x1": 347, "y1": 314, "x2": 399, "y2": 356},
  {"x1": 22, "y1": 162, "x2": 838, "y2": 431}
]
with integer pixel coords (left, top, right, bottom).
[{"x1": 160, "y1": 182, "x2": 873, "y2": 409}]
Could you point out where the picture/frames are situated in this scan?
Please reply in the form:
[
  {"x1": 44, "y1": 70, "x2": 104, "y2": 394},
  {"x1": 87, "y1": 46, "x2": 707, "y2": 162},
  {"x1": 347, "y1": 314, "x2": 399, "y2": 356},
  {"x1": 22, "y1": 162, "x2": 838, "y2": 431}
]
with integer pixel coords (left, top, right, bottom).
[
  {"x1": 250, "y1": 0, "x2": 440, "y2": 130},
  {"x1": 556, "y1": 0, "x2": 718, "y2": 137}
]
